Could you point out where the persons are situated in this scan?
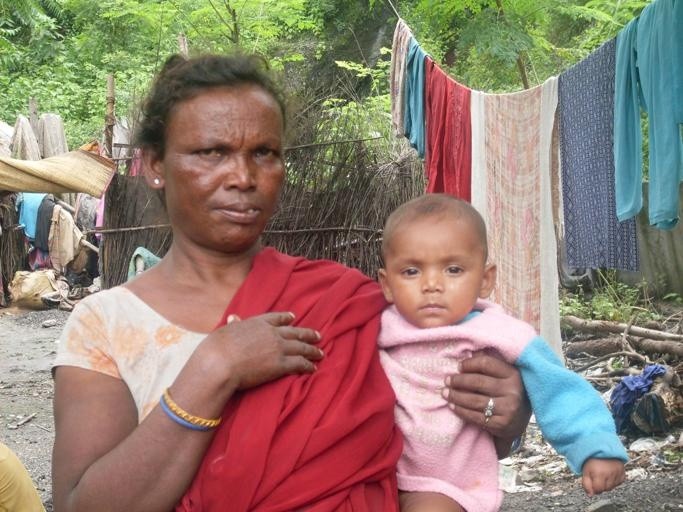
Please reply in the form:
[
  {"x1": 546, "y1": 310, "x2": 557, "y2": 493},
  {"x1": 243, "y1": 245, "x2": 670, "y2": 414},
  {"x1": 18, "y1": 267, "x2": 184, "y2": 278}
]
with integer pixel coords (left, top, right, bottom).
[
  {"x1": 375, "y1": 192, "x2": 628, "y2": 512},
  {"x1": 48, "y1": 51, "x2": 535, "y2": 512}
]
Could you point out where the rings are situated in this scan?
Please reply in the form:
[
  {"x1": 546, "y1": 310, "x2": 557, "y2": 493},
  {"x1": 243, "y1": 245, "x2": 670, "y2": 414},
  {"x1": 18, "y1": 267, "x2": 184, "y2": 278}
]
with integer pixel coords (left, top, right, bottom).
[{"x1": 483, "y1": 398, "x2": 495, "y2": 424}]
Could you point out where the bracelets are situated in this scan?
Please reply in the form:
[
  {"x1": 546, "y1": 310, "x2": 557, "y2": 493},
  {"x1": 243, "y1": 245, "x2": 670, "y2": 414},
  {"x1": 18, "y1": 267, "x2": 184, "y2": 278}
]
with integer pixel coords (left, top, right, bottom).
[
  {"x1": 163, "y1": 389, "x2": 222, "y2": 427},
  {"x1": 159, "y1": 395, "x2": 218, "y2": 432}
]
